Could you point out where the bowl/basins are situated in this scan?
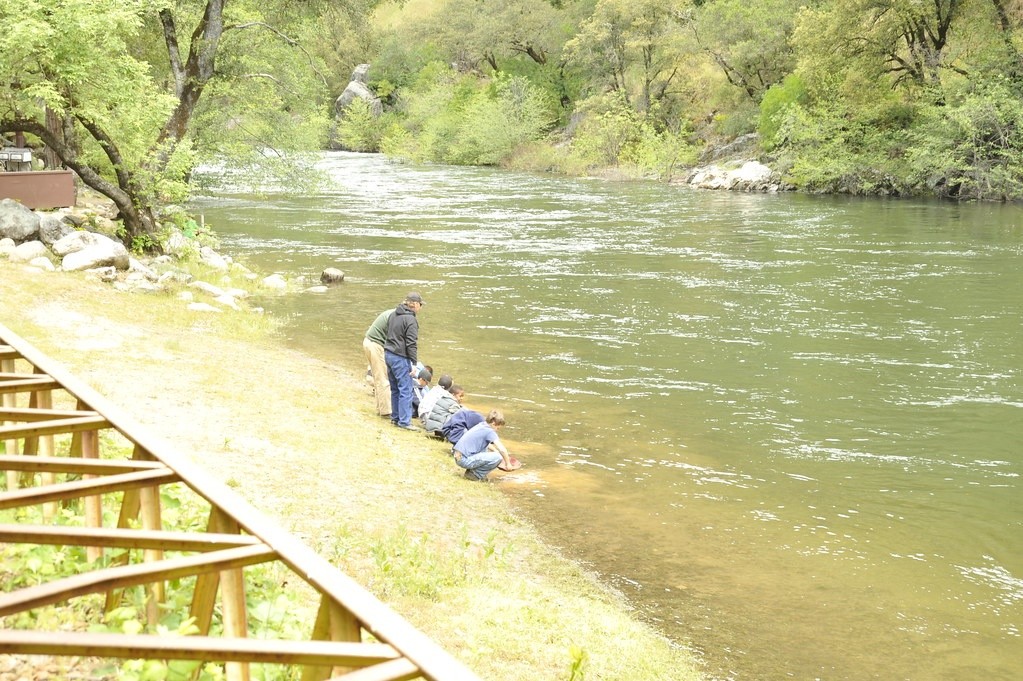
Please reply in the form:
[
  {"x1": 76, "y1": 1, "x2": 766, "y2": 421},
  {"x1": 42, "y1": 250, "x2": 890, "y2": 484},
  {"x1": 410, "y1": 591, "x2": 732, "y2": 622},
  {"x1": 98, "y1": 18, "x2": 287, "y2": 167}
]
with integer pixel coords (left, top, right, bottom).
[{"x1": 497, "y1": 457, "x2": 522, "y2": 472}]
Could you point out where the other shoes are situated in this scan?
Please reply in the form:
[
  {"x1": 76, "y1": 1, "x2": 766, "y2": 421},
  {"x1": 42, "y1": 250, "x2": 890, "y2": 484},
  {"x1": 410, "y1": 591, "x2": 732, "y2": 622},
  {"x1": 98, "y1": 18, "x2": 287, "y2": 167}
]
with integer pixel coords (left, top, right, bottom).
[
  {"x1": 380, "y1": 414, "x2": 392, "y2": 419},
  {"x1": 403, "y1": 425, "x2": 419, "y2": 431},
  {"x1": 464, "y1": 471, "x2": 488, "y2": 482},
  {"x1": 391, "y1": 420, "x2": 398, "y2": 424}
]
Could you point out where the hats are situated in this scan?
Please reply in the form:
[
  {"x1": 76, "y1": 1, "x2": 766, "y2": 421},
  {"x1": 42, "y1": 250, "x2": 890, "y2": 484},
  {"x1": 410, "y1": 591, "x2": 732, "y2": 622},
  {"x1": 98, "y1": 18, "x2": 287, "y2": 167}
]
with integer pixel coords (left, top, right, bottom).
[
  {"x1": 438, "y1": 375, "x2": 452, "y2": 389},
  {"x1": 407, "y1": 293, "x2": 426, "y2": 305},
  {"x1": 418, "y1": 370, "x2": 431, "y2": 382}
]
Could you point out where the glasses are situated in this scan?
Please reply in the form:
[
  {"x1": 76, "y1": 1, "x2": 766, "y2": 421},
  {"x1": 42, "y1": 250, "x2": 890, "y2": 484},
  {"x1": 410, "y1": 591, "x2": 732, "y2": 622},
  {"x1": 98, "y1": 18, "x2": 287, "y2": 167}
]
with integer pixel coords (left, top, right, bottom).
[{"x1": 423, "y1": 379, "x2": 428, "y2": 385}]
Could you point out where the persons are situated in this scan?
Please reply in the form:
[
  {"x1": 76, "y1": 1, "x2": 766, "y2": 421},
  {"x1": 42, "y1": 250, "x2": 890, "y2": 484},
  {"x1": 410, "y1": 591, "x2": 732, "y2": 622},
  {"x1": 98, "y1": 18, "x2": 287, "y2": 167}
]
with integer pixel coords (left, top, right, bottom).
[
  {"x1": 425, "y1": 385, "x2": 464, "y2": 433},
  {"x1": 367, "y1": 365, "x2": 375, "y2": 394},
  {"x1": 418, "y1": 375, "x2": 454, "y2": 425},
  {"x1": 385, "y1": 292, "x2": 426, "y2": 431},
  {"x1": 412, "y1": 370, "x2": 431, "y2": 418},
  {"x1": 362, "y1": 308, "x2": 396, "y2": 420},
  {"x1": 452, "y1": 409, "x2": 512, "y2": 482},
  {"x1": 442, "y1": 406, "x2": 484, "y2": 453},
  {"x1": 411, "y1": 362, "x2": 432, "y2": 379}
]
